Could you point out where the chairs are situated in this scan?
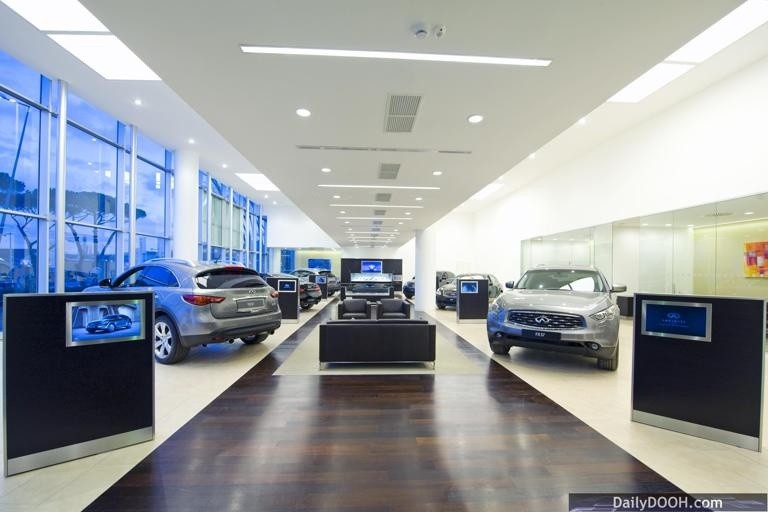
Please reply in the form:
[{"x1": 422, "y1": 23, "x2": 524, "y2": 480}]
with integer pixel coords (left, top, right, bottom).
[{"x1": 337, "y1": 298, "x2": 410, "y2": 320}]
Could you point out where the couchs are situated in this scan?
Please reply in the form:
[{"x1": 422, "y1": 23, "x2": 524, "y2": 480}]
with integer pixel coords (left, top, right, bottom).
[{"x1": 317, "y1": 319, "x2": 436, "y2": 366}]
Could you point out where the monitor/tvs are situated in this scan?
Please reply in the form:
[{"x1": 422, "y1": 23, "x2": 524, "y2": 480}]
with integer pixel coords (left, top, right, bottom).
[
  {"x1": 278, "y1": 280, "x2": 296, "y2": 292},
  {"x1": 64, "y1": 299, "x2": 146, "y2": 347},
  {"x1": 461, "y1": 281, "x2": 479, "y2": 294},
  {"x1": 361, "y1": 261, "x2": 382, "y2": 274},
  {"x1": 316, "y1": 276, "x2": 326, "y2": 283},
  {"x1": 641, "y1": 300, "x2": 713, "y2": 343}
]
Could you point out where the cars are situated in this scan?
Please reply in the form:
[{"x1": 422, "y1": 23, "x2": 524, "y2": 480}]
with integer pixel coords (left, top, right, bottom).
[
  {"x1": 86, "y1": 314, "x2": 132, "y2": 333},
  {"x1": 262, "y1": 269, "x2": 323, "y2": 311}
]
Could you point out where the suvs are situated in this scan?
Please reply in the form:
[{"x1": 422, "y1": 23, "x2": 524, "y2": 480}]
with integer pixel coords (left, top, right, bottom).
[
  {"x1": 485, "y1": 262, "x2": 627, "y2": 371},
  {"x1": 403, "y1": 269, "x2": 502, "y2": 312},
  {"x1": 80, "y1": 256, "x2": 282, "y2": 364},
  {"x1": 289, "y1": 266, "x2": 340, "y2": 298}
]
